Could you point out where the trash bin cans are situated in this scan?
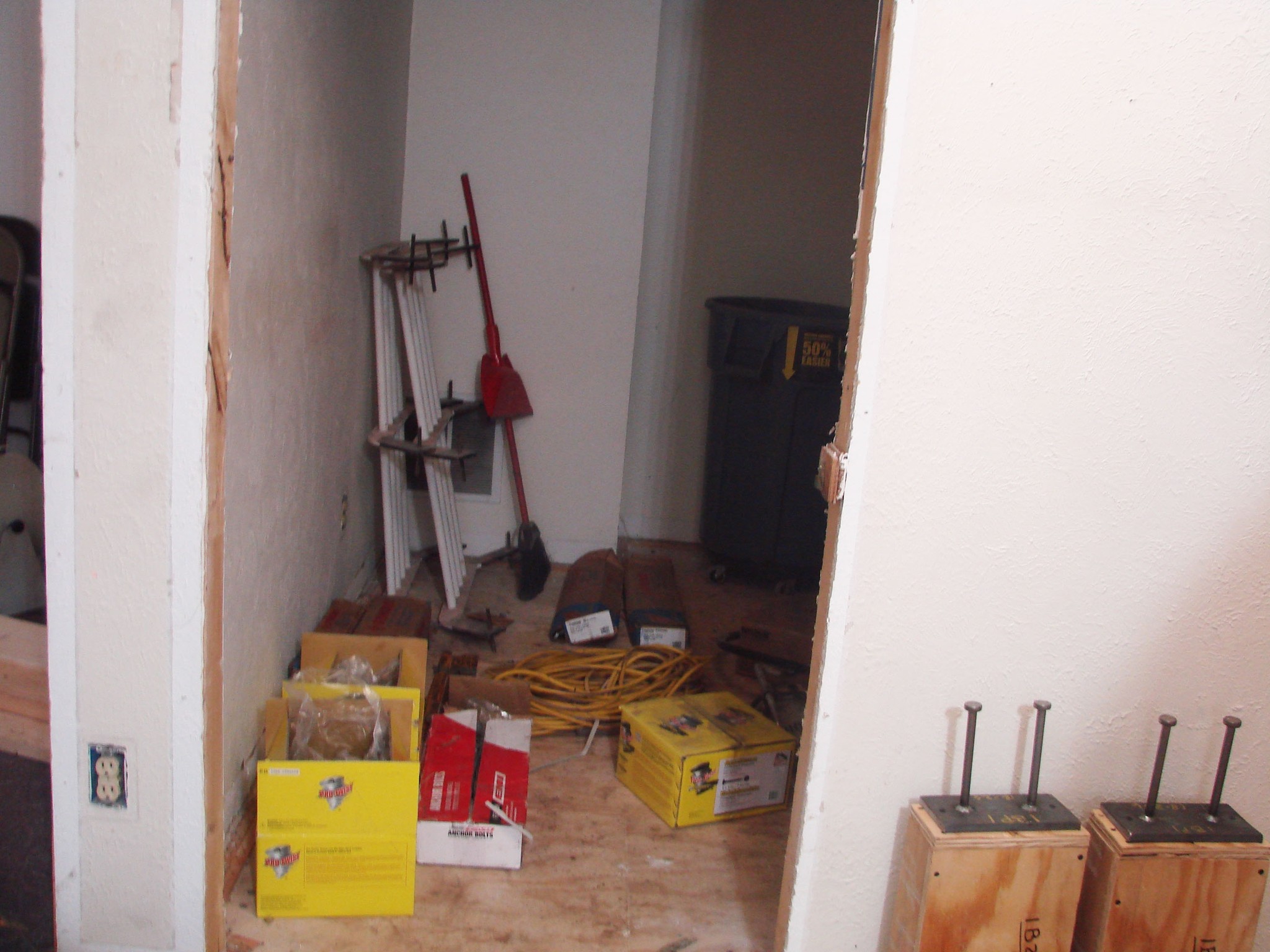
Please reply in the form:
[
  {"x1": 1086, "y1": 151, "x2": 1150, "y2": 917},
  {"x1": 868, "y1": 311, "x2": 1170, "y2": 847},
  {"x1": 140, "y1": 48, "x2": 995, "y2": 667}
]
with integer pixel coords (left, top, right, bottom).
[{"x1": 699, "y1": 296, "x2": 850, "y2": 582}]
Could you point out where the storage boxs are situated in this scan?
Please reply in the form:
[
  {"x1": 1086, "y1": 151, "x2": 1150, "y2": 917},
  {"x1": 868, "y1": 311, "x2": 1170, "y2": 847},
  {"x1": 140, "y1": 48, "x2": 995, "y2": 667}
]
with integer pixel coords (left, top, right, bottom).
[
  {"x1": 614, "y1": 687, "x2": 796, "y2": 830},
  {"x1": 549, "y1": 548, "x2": 622, "y2": 641},
  {"x1": 257, "y1": 595, "x2": 533, "y2": 918},
  {"x1": 621, "y1": 553, "x2": 691, "y2": 653}
]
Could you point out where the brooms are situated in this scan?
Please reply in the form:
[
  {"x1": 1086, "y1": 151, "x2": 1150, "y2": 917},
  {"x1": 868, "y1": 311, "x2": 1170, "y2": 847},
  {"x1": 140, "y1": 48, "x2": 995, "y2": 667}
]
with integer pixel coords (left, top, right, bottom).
[{"x1": 458, "y1": 168, "x2": 554, "y2": 604}]
